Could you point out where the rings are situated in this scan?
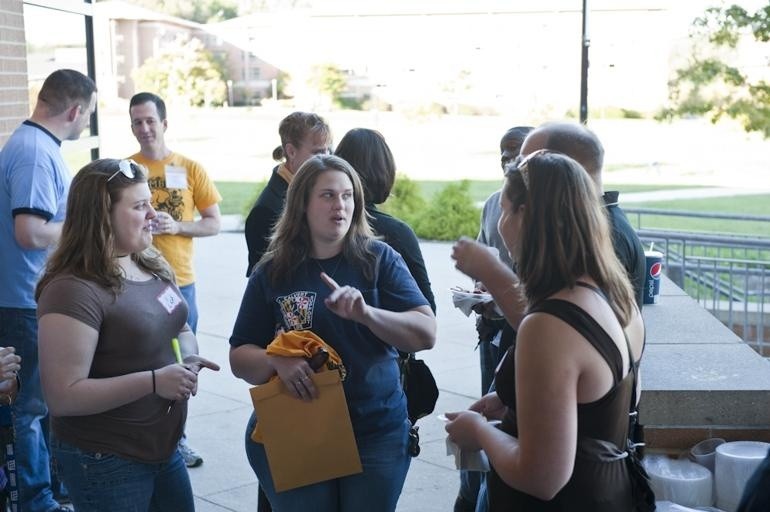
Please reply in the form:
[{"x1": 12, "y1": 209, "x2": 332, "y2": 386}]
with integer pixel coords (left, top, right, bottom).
[
  {"x1": 301, "y1": 377, "x2": 308, "y2": 383},
  {"x1": 155, "y1": 223, "x2": 158, "y2": 228},
  {"x1": 296, "y1": 382, "x2": 301, "y2": 385},
  {"x1": 8, "y1": 394, "x2": 13, "y2": 406}
]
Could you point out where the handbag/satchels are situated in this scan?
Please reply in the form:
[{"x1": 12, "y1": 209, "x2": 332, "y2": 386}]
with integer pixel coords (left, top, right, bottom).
[{"x1": 627, "y1": 458, "x2": 656, "y2": 512}]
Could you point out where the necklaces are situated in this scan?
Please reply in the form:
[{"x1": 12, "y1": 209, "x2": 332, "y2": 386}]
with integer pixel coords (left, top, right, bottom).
[{"x1": 309, "y1": 251, "x2": 343, "y2": 281}]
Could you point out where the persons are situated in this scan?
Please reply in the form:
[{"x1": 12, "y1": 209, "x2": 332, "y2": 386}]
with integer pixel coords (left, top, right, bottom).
[
  {"x1": 0, "y1": 342, "x2": 32, "y2": 511},
  {"x1": 336, "y1": 129, "x2": 440, "y2": 456},
  {"x1": 1, "y1": 67, "x2": 100, "y2": 510},
  {"x1": 452, "y1": 124, "x2": 645, "y2": 403},
  {"x1": 445, "y1": 147, "x2": 665, "y2": 509},
  {"x1": 229, "y1": 152, "x2": 438, "y2": 511},
  {"x1": 243, "y1": 110, "x2": 334, "y2": 511},
  {"x1": 451, "y1": 124, "x2": 541, "y2": 509},
  {"x1": 33, "y1": 157, "x2": 221, "y2": 511},
  {"x1": 112, "y1": 90, "x2": 219, "y2": 469}
]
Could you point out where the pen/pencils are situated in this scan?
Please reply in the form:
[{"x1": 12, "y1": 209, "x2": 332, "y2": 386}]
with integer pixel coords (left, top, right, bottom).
[
  {"x1": 172, "y1": 338, "x2": 183, "y2": 364},
  {"x1": 167, "y1": 367, "x2": 191, "y2": 412}
]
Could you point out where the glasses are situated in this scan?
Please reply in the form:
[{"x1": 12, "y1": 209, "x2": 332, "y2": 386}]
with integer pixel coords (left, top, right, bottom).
[
  {"x1": 106, "y1": 159, "x2": 147, "y2": 183},
  {"x1": 518, "y1": 147, "x2": 551, "y2": 191}
]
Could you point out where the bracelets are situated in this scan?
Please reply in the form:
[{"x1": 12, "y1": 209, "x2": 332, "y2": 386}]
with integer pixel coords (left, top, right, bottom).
[
  {"x1": 150, "y1": 366, "x2": 158, "y2": 398},
  {"x1": 14, "y1": 371, "x2": 21, "y2": 393}
]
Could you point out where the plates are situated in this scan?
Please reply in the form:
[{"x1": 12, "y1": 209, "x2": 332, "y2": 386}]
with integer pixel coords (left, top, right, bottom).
[
  {"x1": 451, "y1": 290, "x2": 492, "y2": 299},
  {"x1": 437, "y1": 412, "x2": 503, "y2": 427}
]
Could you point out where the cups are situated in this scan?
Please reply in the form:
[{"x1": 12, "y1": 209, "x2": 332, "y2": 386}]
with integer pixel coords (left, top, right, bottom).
[
  {"x1": 686, "y1": 437, "x2": 727, "y2": 474},
  {"x1": 641, "y1": 250, "x2": 664, "y2": 304},
  {"x1": 150, "y1": 213, "x2": 164, "y2": 234}
]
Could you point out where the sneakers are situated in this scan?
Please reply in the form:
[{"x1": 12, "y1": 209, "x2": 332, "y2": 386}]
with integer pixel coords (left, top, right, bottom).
[{"x1": 177, "y1": 434, "x2": 202, "y2": 468}]
